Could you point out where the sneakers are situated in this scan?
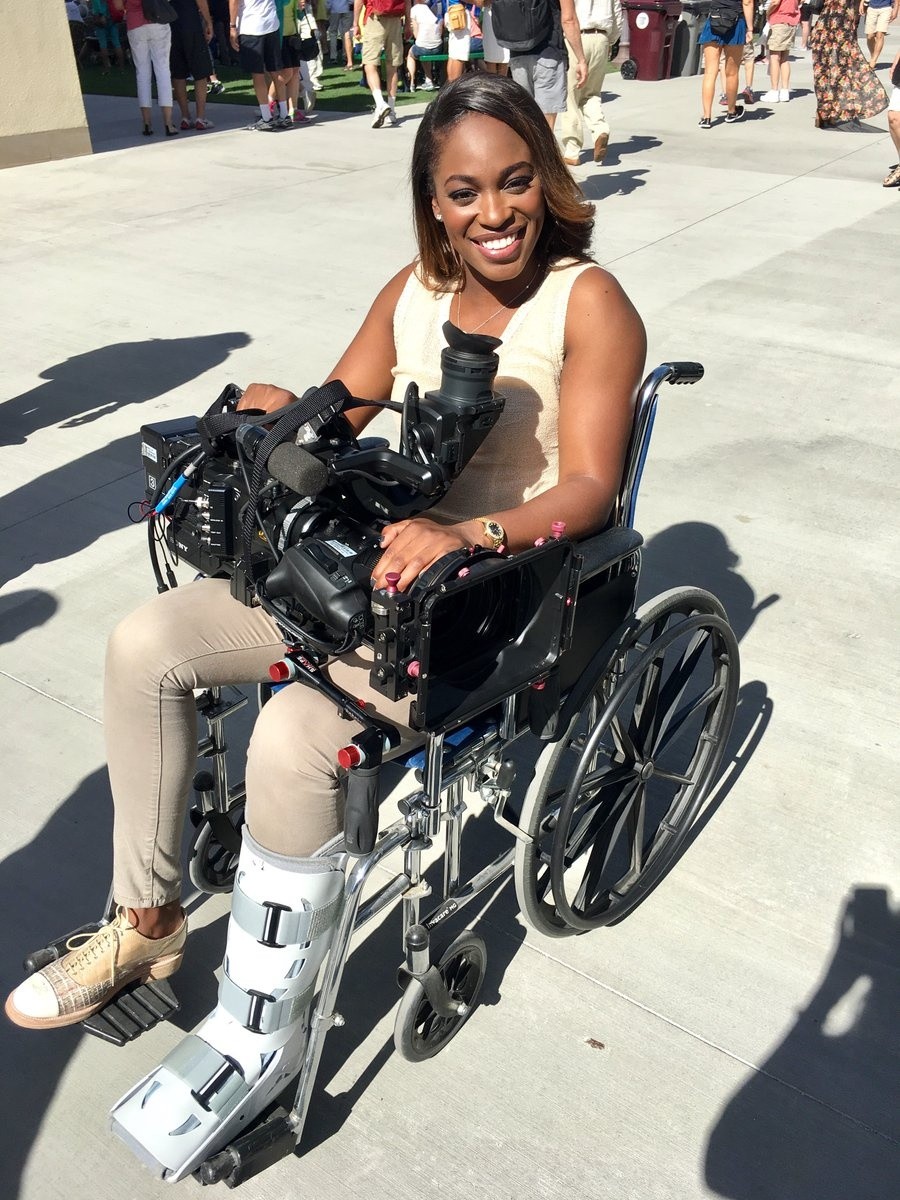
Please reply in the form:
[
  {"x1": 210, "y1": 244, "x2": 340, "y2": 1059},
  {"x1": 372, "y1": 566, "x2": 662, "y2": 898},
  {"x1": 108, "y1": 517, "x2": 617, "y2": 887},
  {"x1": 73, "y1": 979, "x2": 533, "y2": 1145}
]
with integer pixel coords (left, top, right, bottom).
[
  {"x1": 194, "y1": 117, "x2": 215, "y2": 130},
  {"x1": 255, "y1": 114, "x2": 296, "y2": 129},
  {"x1": 180, "y1": 117, "x2": 195, "y2": 130},
  {"x1": 385, "y1": 116, "x2": 397, "y2": 124},
  {"x1": 725, "y1": 105, "x2": 745, "y2": 122},
  {"x1": 698, "y1": 118, "x2": 711, "y2": 128},
  {"x1": 779, "y1": 89, "x2": 790, "y2": 102},
  {"x1": 371, "y1": 103, "x2": 392, "y2": 128},
  {"x1": 4, "y1": 905, "x2": 189, "y2": 1030},
  {"x1": 248, "y1": 118, "x2": 277, "y2": 132},
  {"x1": 742, "y1": 88, "x2": 754, "y2": 104},
  {"x1": 760, "y1": 89, "x2": 780, "y2": 103}
]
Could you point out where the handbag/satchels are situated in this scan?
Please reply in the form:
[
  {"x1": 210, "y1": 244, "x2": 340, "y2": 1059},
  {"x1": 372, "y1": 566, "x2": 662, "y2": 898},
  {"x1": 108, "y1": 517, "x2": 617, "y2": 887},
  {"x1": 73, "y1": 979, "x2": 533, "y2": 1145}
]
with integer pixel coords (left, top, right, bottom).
[
  {"x1": 299, "y1": 37, "x2": 319, "y2": 61},
  {"x1": 448, "y1": 4, "x2": 466, "y2": 31},
  {"x1": 710, "y1": 9, "x2": 739, "y2": 35}
]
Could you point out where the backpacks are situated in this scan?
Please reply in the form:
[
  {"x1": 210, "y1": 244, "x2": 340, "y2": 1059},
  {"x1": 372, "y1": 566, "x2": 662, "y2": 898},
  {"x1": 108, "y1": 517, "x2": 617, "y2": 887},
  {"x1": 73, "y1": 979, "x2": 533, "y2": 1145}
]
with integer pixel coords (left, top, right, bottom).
[
  {"x1": 490, "y1": 1, "x2": 553, "y2": 51},
  {"x1": 367, "y1": 0, "x2": 407, "y2": 18}
]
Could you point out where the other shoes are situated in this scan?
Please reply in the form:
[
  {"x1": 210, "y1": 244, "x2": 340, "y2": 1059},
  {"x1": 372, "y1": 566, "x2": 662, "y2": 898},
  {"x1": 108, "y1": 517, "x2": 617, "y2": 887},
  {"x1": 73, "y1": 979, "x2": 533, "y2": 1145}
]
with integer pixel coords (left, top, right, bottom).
[
  {"x1": 209, "y1": 81, "x2": 225, "y2": 96},
  {"x1": 719, "y1": 97, "x2": 728, "y2": 105},
  {"x1": 269, "y1": 100, "x2": 277, "y2": 116},
  {"x1": 165, "y1": 124, "x2": 179, "y2": 136},
  {"x1": 594, "y1": 133, "x2": 608, "y2": 162},
  {"x1": 797, "y1": 46, "x2": 808, "y2": 51},
  {"x1": 313, "y1": 83, "x2": 324, "y2": 91},
  {"x1": 883, "y1": 165, "x2": 900, "y2": 186},
  {"x1": 404, "y1": 84, "x2": 415, "y2": 93},
  {"x1": 421, "y1": 82, "x2": 434, "y2": 92},
  {"x1": 288, "y1": 110, "x2": 311, "y2": 124},
  {"x1": 142, "y1": 123, "x2": 153, "y2": 136},
  {"x1": 564, "y1": 158, "x2": 581, "y2": 166},
  {"x1": 359, "y1": 79, "x2": 369, "y2": 89},
  {"x1": 344, "y1": 66, "x2": 353, "y2": 71}
]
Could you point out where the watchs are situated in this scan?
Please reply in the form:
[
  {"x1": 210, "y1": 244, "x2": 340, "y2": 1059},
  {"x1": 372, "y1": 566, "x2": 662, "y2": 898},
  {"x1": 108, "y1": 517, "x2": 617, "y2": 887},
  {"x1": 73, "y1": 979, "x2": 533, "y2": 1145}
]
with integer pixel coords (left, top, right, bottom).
[{"x1": 473, "y1": 517, "x2": 505, "y2": 552}]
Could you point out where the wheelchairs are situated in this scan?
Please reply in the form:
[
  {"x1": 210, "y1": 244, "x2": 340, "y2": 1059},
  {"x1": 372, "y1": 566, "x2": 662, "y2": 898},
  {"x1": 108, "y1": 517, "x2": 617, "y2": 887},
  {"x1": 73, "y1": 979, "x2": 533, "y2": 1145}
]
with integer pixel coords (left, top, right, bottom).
[{"x1": 22, "y1": 321, "x2": 739, "y2": 1191}]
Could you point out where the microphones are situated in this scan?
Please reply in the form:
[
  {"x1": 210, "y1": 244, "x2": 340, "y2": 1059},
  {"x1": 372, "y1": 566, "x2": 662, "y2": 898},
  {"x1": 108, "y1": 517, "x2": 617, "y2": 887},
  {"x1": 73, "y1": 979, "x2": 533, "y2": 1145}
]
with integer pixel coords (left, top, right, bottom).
[{"x1": 235, "y1": 422, "x2": 331, "y2": 497}]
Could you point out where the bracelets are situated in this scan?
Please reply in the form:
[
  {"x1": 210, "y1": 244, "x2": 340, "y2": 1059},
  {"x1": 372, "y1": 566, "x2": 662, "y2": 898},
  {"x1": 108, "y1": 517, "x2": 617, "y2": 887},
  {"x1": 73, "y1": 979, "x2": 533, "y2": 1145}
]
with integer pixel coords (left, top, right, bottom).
[{"x1": 230, "y1": 25, "x2": 236, "y2": 28}]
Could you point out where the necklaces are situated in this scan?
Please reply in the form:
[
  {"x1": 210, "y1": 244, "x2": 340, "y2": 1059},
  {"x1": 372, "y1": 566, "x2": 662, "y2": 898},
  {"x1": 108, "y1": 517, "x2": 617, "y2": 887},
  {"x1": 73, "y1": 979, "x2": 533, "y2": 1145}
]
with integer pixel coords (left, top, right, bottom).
[{"x1": 457, "y1": 252, "x2": 542, "y2": 331}]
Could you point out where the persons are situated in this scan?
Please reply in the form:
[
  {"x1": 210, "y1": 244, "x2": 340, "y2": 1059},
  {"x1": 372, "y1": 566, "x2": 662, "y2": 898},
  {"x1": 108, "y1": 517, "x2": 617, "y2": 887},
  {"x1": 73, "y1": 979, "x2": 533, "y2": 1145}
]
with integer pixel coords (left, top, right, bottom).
[
  {"x1": 2, "y1": 71, "x2": 648, "y2": 1188},
  {"x1": 65, "y1": 0, "x2": 623, "y2": 167}
]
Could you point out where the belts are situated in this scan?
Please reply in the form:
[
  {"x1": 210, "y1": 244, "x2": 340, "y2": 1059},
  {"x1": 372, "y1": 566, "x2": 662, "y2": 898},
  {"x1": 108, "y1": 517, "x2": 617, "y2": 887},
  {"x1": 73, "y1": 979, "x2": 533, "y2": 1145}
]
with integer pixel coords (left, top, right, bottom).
[{"x1": 581, "y1": 28, "x2": 606, "y2": 34}]
